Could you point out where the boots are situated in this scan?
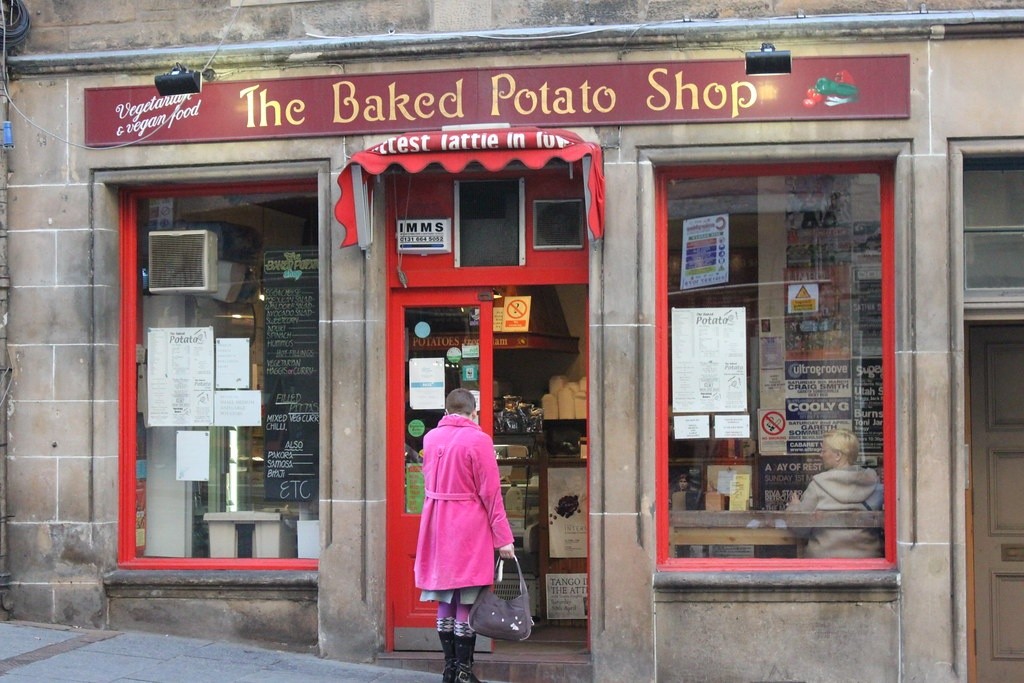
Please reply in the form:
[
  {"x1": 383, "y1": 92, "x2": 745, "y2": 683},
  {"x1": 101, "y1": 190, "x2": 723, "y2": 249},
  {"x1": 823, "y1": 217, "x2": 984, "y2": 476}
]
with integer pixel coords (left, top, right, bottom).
[
  {"x1": 453, "y1": 631, "x2": 480, "y2": 683},
  {"x1": 437, "y1": 629, "x2": 457, "y2": 683}
]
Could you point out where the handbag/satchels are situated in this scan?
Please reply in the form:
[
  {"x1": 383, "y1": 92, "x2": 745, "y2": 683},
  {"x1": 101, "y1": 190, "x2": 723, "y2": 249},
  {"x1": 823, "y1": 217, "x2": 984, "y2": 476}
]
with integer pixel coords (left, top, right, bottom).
[{"x1": 467, "y1": 554, "x2": 531, "y2": 640}]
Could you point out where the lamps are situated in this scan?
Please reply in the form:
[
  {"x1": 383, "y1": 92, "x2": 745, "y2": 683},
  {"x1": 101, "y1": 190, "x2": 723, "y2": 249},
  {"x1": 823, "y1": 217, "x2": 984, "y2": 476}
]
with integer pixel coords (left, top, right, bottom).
[
  {"x1": 745, "y1": 42, "x2": 792, "y2": 75},
  {"x1": 154, "y1": 62, "x2": 216, "y2": 97}
]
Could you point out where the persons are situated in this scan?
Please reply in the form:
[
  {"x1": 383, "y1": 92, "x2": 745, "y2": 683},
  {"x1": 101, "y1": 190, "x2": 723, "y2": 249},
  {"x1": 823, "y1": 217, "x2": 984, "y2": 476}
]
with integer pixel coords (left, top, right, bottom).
[
  {"x1": 784, "y1": 428, "x2": 885, "y2": 558},
  {"x1": 413, "y1": 387, "x2": 514, "y2": 682},
  {"x1": 668, "y1": 474, "x2": 695, "y2": 511}
]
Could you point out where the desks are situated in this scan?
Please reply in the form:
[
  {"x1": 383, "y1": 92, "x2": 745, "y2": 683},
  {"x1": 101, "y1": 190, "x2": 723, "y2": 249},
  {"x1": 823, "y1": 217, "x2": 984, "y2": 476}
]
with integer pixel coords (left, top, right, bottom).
[
  {"x1": 202, "y1": 512, "x2": 297, "y2": 559},
  {"x1": 669, "y1": 528, "x2": 809, "y2": 558}
]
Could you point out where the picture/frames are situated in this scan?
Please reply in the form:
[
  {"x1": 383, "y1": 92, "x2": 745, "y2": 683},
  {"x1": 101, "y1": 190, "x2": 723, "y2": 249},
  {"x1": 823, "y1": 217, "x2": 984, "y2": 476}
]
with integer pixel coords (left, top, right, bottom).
[{"x1": 214, "y1": 335, "x2": 252, "y2": 390}]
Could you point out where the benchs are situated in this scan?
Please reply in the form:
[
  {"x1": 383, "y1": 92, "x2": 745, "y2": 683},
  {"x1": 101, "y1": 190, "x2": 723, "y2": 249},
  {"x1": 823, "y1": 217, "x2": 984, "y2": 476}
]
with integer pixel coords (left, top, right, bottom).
[{"x1": 669, "y1": 510, "x2": 884, "y2": 558}]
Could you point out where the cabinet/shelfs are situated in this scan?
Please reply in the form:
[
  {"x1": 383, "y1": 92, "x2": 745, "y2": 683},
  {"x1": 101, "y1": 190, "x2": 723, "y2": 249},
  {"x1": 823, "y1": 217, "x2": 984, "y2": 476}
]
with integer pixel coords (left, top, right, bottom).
[{"x1": 494, "y1": 432, "x2": 540, "y2": 554}]
